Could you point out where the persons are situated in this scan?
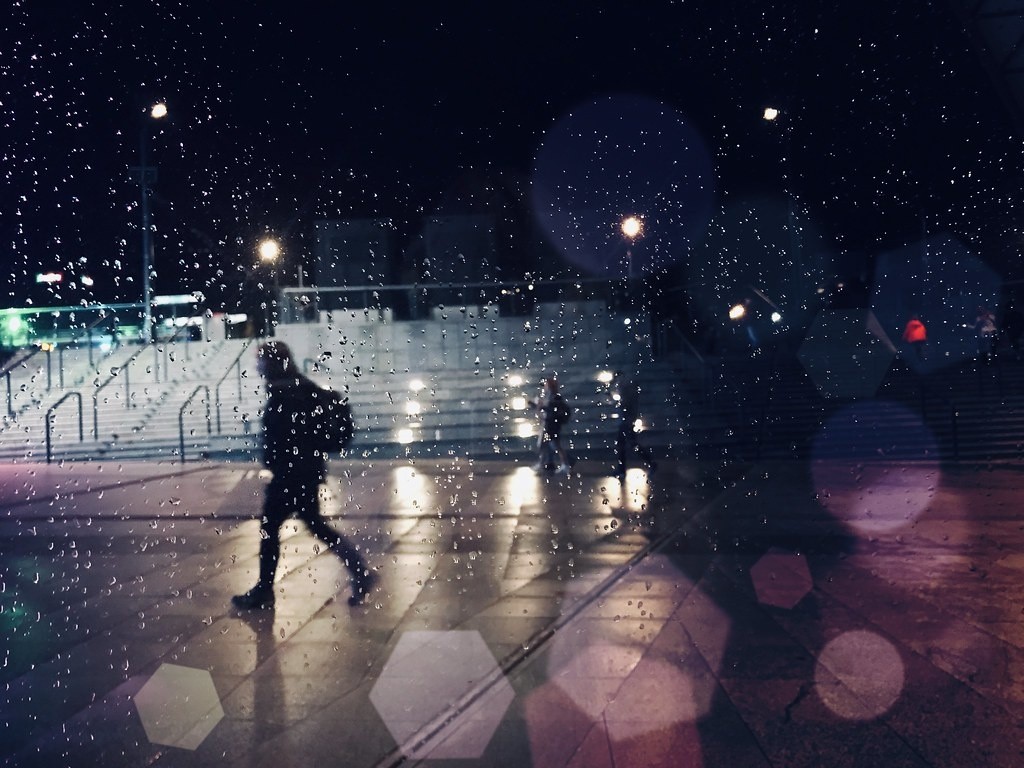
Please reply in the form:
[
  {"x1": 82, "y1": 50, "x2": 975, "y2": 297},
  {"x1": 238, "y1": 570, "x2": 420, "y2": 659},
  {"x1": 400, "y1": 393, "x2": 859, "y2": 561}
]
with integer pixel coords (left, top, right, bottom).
[
  {"x1": 610, "y1": 387, "x2": 659, "y2": 482},
  {"x1": 528, "y1": 378, "x2": 574, "y2": 476},
  {"x1": 232, "y1": 340, "x2": 378, "y2": 614}
]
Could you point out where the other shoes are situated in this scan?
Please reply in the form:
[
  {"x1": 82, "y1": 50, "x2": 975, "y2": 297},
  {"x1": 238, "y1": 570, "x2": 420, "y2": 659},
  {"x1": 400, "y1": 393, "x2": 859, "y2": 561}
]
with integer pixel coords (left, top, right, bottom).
[
  {"x1": 545, "y1": 462, "x2": 557, "y2": 471},
  {"x1": 565, "y1": 455, "x2": 576, "y2": 466},
  {"x1": 348, "y1": 570, "x2": 377, "y2": 606},
  {"x1": 555, "y1": 462, "x2": 571, "y2": 474},
  {"x1": 231, "y1": 591, "x2": 276, "y2": 610},
  {"x1": 533, "y1": 463, "x2": 544, "y2": 470},
  {"x1": 610, "y1": 468, "x2": 627, "y2": 479},
  {"x1": 647, "y1": 461, "x2": 658, "y2": 476}
]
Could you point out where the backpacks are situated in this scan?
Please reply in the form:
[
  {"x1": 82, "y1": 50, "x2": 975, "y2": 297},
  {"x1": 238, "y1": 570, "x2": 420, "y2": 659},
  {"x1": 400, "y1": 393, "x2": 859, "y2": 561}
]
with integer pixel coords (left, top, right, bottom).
[{"x1": 315, "y1": 387, "x2": 354, "y2": 453}]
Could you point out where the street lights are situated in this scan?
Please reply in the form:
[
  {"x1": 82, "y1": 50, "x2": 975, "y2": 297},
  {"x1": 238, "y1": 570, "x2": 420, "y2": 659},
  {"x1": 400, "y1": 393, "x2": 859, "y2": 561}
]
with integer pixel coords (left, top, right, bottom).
[
  {"x1": 621, "y1": 215, "x2": 644, "y2": 314},
  {"x1": 135, "y1": 96, "x2": 170, "y2": 345},
  {"x1": 255, "y1": 237, "x2": 282, "y2": 339}
]
